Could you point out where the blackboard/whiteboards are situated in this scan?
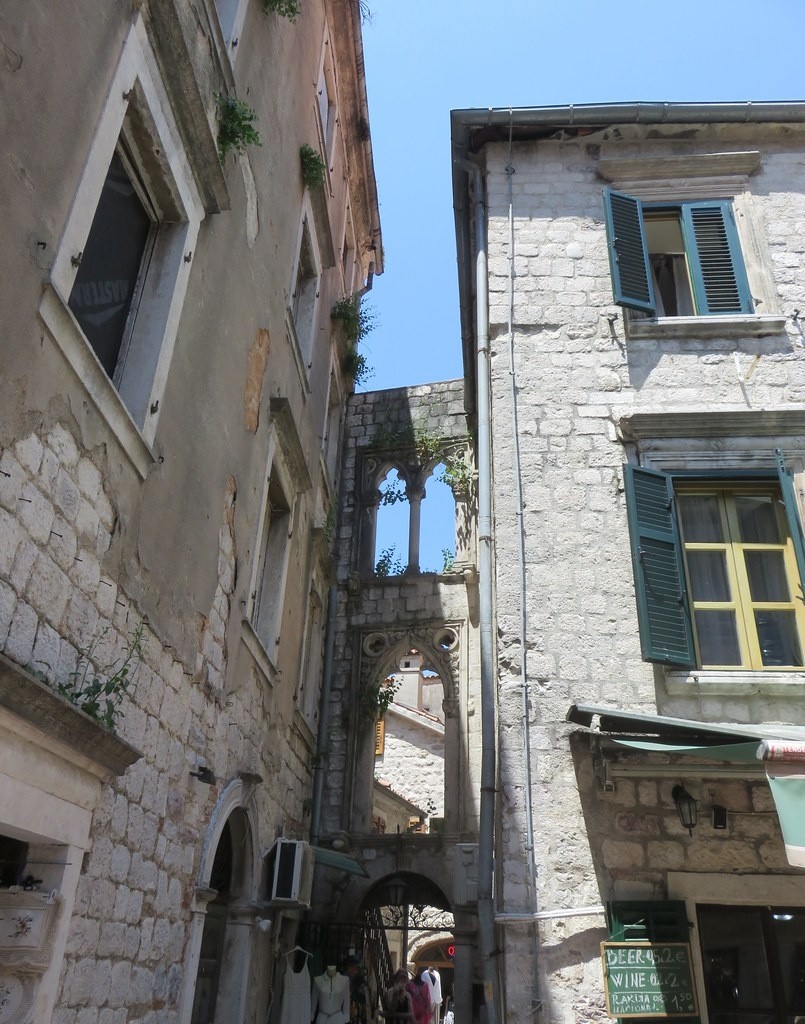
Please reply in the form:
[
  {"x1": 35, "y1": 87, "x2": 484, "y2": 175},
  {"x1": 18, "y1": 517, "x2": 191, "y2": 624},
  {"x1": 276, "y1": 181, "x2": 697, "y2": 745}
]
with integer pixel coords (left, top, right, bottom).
[{"x1": 600, "y1": 942, "x2": 699, "y2": 1018}]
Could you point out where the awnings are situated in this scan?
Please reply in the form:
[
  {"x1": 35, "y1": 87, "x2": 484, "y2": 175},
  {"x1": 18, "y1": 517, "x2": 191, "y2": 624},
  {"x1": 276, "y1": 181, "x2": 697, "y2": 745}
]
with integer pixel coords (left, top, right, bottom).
[{"x1": 590, "y1": 736, "x2": 805, "y2": 868}]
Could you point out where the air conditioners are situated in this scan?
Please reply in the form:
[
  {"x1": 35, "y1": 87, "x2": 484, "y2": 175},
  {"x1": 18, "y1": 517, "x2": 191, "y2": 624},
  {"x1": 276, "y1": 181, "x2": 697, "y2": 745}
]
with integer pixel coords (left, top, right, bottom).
[{"x1": 271, "y1": 840, "x2": 317, "y2": 907}]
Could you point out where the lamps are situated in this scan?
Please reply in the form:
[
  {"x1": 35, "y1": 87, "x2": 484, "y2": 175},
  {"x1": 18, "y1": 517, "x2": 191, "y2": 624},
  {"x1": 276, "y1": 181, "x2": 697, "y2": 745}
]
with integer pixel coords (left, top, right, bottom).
[{"x1": 672, "y1": 784, "x2": 700, "y2": 837}]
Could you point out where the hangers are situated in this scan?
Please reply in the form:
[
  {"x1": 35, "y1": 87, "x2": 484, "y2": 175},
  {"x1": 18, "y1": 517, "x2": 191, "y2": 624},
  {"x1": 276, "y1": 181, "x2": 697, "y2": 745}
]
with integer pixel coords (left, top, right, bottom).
[{"x1": 282, "y1": 941, "x2": 314, "y2": 958}]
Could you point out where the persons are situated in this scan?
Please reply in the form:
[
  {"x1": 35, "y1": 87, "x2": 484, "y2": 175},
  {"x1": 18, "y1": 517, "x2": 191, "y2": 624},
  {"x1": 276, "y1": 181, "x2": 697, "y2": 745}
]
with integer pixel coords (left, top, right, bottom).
[
  {"x1": 312, "y1": 966, "x2": 350, "y2": 1024},
  {"x1": 383, "y1": 968, "x2": 417, "y2": 1024},
  {"x1": 408, "y1": 966, "x2": 443, "y2": 1024}
]
